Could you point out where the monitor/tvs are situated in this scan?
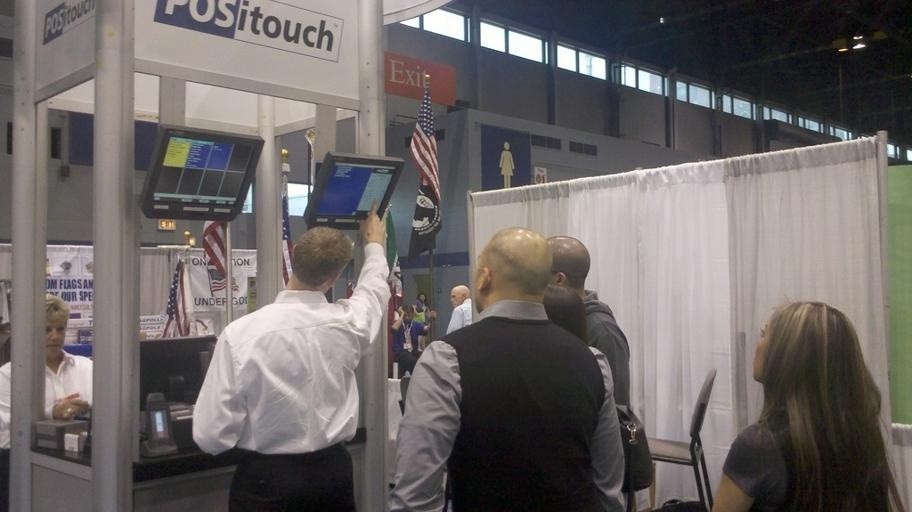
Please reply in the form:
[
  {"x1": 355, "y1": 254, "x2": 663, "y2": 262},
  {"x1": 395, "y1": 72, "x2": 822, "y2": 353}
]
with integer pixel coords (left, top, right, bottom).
[
  {"x1": 302, "y1": 151, "x2": 405, "y2": 230},
  {"x1": 139, "y1": 335, "x2": 217, "y2": 410},
  {"x1": 139, "y1": 124, "x2": 266, "y2": 221}
]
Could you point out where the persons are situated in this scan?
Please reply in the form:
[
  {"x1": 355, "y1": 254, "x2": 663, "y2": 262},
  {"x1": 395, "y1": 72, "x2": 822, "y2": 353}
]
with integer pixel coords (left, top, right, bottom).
[
  {"x1": 0, "y1": 292, "x2": 94, "y2": 452},
  {"x1": 709, "y1": 300, "x2": 893, "y2": 510},
  {"x1": 390, "y1": 282, "x2": 473, "y2": 379},
  {"x1": 388, "y1": 225, "x2": 629, "y2": 511},
  {"x1": 539, "y1": 236, "x2": 631, "y2": 510},
  {"x1": 541, "y1": 281, "x2": 590, "y2": 347},
  {"x1": 191, "y1": 194, "x2": 393, "y2": 511}
]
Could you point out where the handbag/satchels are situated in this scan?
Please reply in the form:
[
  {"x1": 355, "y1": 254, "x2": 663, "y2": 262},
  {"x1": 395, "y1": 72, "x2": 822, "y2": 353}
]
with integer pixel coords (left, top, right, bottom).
[{"x1": 612, "y1": 400, "x2": 659, "y2": 496}]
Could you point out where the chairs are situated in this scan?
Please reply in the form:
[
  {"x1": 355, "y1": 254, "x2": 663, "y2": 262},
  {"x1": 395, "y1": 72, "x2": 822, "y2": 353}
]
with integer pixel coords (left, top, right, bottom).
[{"x1": 643, "y1": 370, "x2": 714, "y2": 512}]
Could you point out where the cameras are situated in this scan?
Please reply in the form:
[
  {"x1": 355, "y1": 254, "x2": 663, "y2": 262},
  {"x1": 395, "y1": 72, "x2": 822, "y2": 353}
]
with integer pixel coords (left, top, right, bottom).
[{"x1": 402, "y1": 304, "x2": 412, "y2": 313}]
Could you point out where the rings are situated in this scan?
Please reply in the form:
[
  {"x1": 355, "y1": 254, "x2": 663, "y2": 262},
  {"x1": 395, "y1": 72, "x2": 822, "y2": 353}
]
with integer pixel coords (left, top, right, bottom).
[{"x1": 66, "y1": 407, "x2": 73, "y2": 413}]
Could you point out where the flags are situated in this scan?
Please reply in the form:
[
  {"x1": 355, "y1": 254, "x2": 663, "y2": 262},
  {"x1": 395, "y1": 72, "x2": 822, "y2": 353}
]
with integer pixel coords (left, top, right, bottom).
[
  {"x1": 345, "y1": 202, "x2": 404, "y2": 348},
  {"x1": 165, "y1": 255, "x2": 193, "y2": 337},
  {"x1": 407, "y1": 77, "x2": 445, "y2": 264},
  {"x1": 281, "y1": 171, "x2": 295, "y2": 293},
  {"x1": 201, "y1": 216, "x2": 242, "y2": 299}
]
